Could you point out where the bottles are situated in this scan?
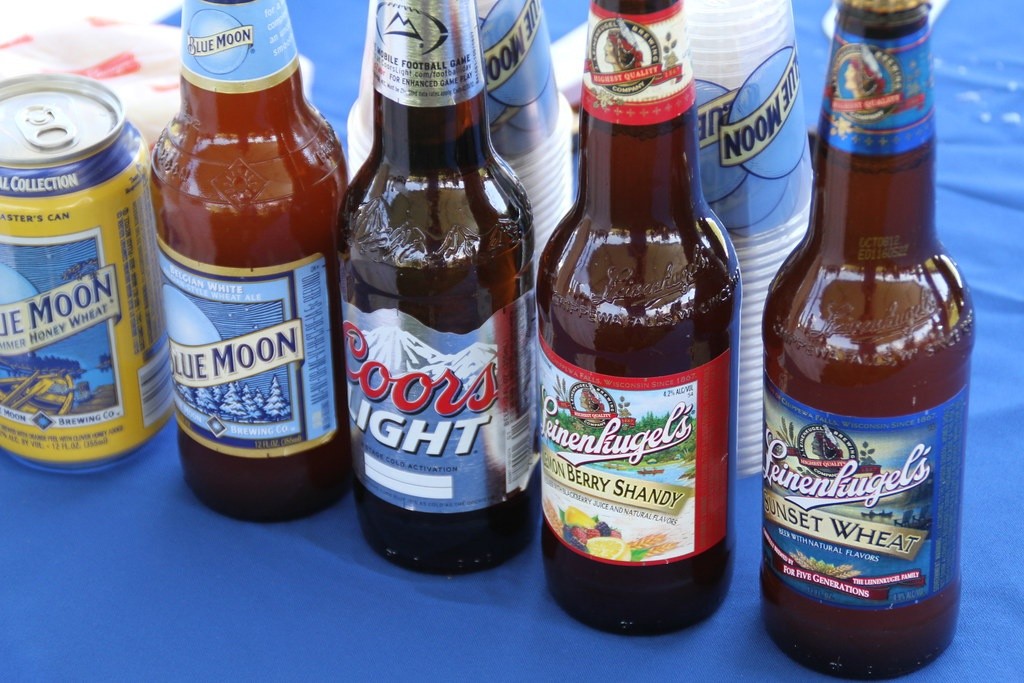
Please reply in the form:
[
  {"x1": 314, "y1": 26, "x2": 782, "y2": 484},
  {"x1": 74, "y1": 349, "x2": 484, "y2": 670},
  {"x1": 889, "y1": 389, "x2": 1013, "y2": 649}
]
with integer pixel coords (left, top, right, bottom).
[
  {"x1": 149, "y1": 0, "x2": 350, "y2": 522},
  {"x1": 338, "y1": 1, "x2": 539, "y2": 575},
  {"x1": 762, "y1": 1, "x2": 975, "y2": 680}
]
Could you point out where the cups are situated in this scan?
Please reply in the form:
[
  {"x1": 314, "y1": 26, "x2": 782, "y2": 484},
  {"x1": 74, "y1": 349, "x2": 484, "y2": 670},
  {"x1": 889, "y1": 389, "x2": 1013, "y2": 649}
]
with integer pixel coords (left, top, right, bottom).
[
  {"x1": 536, "y1": 0, "x2": 743, "y2": 635},
  {"x1": 345, "y1": 0, "x2": 576, "y2": 271},
  {"x1": 686, "y1": 1, "x2": 815, "y2": 477}
]
